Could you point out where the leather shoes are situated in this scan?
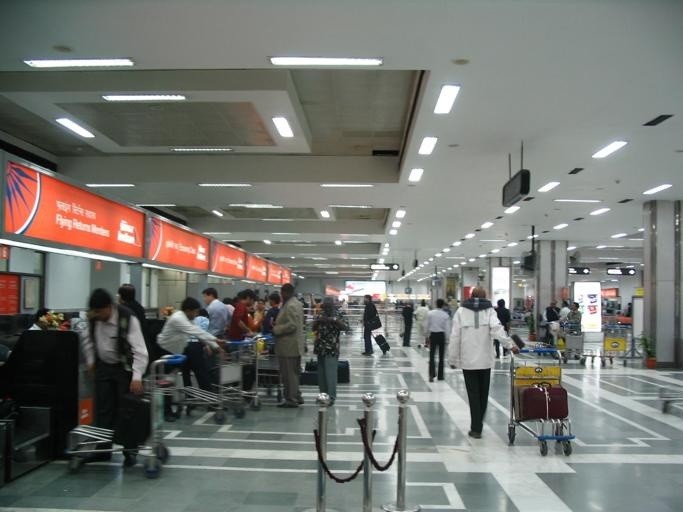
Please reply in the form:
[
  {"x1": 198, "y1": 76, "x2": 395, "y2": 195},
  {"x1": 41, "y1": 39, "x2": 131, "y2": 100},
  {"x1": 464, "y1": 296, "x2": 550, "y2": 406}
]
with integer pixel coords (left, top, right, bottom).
[
  {"x1": 274, "y1": 397, "x2": 334, "y2": 409},
  {"x1": 83, "y1": 446, "x2": 139, "y2": 467},
  {"x1": 468, "y1": 429, "x2": 482, "y2": 439}
]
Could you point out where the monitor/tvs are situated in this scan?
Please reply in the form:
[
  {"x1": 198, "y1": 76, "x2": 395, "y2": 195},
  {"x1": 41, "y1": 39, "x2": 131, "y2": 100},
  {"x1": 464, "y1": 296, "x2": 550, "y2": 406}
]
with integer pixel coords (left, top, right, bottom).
[
  {"x1": 405, "y1": 288, "x2": 412, "y2": 293},
  {"x1": 431, "y1": 280, "x2": 441, "y2": 287},
  {"x1": 521, "y1": 251, "x2": 536, "y2": 270}
]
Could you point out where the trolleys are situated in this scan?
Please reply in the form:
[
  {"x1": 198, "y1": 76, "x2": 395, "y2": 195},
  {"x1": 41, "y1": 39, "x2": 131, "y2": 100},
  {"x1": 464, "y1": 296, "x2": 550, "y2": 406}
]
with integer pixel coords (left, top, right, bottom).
[
  {"x1": 503, "y1": 346, "x2": 577, "y2": 457},
  {"x1": 601, "y1": 322, "x2": 632, "y2": 368},
  {"x1": 166, "y1": 335, "x2": 285, "y2": 423},
  {"x1": 62, "y1": 354, "x2": 192, "y2": 485},
  {"x1": 534, "y1": 320, "x2": 559, "y2": 359},
  {"x1": 561, "y1": 322, "x2": 588, "y2": 367}
]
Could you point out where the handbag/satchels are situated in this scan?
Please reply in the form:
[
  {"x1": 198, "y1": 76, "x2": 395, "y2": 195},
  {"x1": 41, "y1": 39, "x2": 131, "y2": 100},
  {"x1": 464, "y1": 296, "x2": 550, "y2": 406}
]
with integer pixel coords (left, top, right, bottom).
[
  {"x1": 366, "y1": 315, "x2": 382, "y2": 331},
  {"x1": 521, "y1": 381, "x2": 569, "y2": 420}
]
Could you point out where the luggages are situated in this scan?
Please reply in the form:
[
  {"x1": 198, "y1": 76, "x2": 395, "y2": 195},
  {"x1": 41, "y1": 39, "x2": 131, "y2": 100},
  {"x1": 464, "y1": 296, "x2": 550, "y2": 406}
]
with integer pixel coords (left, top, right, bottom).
[
  {"x1": 112, "y1": 391, "x2": 153, "y2": 448},
  {"x1": 373, "y1": 334, "x2": 390, "y2": 353},
  {"x1": 510, "y1": 333, "x2": 525, "y2": 349}
]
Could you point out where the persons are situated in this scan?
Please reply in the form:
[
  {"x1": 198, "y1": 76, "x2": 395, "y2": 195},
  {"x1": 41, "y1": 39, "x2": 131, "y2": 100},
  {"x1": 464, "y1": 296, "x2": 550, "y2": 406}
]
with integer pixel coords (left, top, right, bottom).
[
  {"x1": 494, "y1": 300, "x2": 511, "y2": 359},
  {"x1": 564, "y1": 302, "x2": 582, "y2": 359},
  {"x1": 447, "y1": 287, "x2": 520, "y2": 439},
  {"x1": 543, "y1": 300, "x2": 561, "y2": 344},
  {"x1": 557, "y1": 300, "x2": 571, "y2": 321}
]
